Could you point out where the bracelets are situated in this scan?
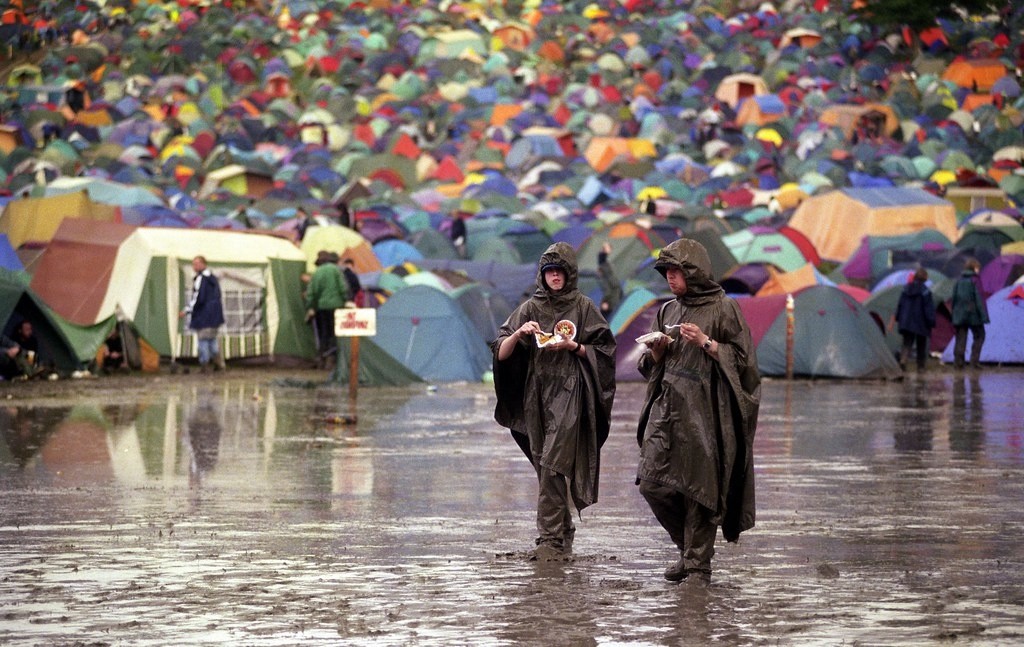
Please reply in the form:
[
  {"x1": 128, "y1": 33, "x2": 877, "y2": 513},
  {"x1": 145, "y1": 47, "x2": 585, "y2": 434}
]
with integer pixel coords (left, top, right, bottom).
[
  {"x1": 702, "y1": 337, "x2": 712, "y2": 350},
  {"x1": 571, "y1": 343, "x2": 581, "y2": 353}
]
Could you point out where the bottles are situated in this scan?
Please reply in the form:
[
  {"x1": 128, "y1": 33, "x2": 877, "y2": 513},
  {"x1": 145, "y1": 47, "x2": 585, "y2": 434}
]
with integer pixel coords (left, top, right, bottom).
[{"x1": 319, "y1": 413, "x2": 358, "y2": 425}]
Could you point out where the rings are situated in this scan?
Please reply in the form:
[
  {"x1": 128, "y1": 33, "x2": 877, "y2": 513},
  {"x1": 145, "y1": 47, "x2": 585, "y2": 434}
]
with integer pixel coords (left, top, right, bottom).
[
  {"x1": 556, "y1": 347, "x2": 558, "y2": 349},
  {"x1": 686, "y1": 335, "x2": 688, "y2": 337}
]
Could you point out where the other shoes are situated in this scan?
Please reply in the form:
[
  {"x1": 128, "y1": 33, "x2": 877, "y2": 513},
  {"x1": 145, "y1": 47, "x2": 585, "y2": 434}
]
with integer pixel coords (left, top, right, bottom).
[
  {"x1": 680, "y1": 575, "x2": 706, "y2": 588},
  {"x1": 664, "y1": 553, "x2": 689, "y2": 580}
]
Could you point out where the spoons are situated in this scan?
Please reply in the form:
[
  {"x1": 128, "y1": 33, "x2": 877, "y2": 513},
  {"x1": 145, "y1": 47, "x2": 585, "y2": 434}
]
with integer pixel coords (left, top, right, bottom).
[
  {"x1": 664, "y1": 324, "x2": 691, "y2": 329},
  {"x1": 535, "y1": 329, "x2": 553, "y2": 336}
]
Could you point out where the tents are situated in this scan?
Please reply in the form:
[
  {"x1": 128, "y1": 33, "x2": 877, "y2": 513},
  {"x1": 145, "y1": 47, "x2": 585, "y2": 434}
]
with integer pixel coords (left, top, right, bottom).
[{"x1": 0, "y1": 0, "x2": 1023, "y2": 386}]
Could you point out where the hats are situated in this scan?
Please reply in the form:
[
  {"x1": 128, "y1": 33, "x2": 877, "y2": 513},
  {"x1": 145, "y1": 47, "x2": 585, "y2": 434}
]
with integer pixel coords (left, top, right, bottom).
[{"x1": 315, "y1": 251, "x2": 337, "y2": 265}]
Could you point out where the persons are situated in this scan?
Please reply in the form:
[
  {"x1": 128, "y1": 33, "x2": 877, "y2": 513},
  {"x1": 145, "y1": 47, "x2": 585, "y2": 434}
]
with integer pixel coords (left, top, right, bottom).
[
  {"x1": 451, "y1": 210, "x2": 467, "y2": 259},
  {"x1": 951, "y1": 258, "x2": 989, "y2": 372},
  {"x1": 292, "y1": 206, "x2": 317, "y2": 241},
  {"x1": 0, "y1": 335, "x2": 25, "y2": 379},
  {"x1": 178, "y1": 256, "x2": 224, "y2": 372},
  {"x1": 331, "y1": 252, "x2": 359, "y2": 302},
  {"x1": 634, "y1": 238, "x2": 761, "y2": 579},
  {"x1": 102, "y1": 323, "x2": 123, "y2": 373},
  {"x1": 16, "y1": 321, "x2": 55, "y2": 368},
  {"x1": 888, "y1": 268, "x2": 936, "y2": 373},
  {"x1": 302, "y1": 251, "x2": 348, "y2": 357},
  {"x1": 336, "y1": 200, "x2": 356, "y2": 229},
  {"x1": 647, "y1": 195, "x2": 655, "y2": 214},
  {"x1": 492, "y1": 243, "x2": 618, "y2": 553}
]
